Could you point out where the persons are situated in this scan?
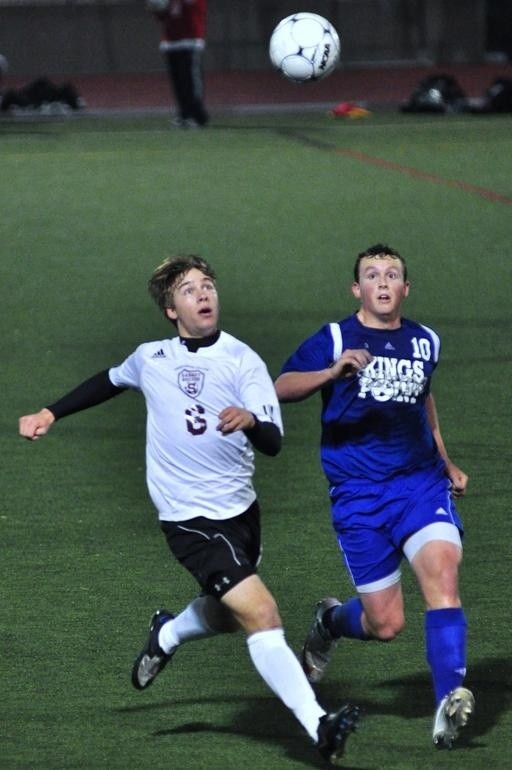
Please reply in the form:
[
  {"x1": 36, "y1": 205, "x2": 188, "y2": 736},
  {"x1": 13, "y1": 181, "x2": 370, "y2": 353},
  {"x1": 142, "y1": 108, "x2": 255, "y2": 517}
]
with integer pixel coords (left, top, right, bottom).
[
  {"x1": 15, "y1": 252, "x2": 368, "y2": 768},
  {"x1": 272, "y1": 241, "x2": 478, "y2": 749},
  {"x1": 148, "y1": 0, "x2": 210, "y2": 129}
]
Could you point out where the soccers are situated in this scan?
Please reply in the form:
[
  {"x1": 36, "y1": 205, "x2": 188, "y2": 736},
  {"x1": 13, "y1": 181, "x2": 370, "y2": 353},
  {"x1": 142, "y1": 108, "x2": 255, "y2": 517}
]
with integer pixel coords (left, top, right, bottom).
[{"x1": 268, "y1": 12, "x2": 343, "y2": 82}]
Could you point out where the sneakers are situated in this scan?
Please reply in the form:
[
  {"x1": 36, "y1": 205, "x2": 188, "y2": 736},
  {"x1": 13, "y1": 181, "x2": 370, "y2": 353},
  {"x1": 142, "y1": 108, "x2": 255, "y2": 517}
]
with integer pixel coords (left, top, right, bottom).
[
  {"x1": 300, "y1": 596, "x2": 346, "y2": 686},
  {"x1": 129, "y1": 607, "x2": 179, "y2": 691},
  {"x1": 431, "y1": 685, "x2": 478, "y2": 752},
  {"x1": 314, "y1": 703, "x2": 362, "y2": 769}
]
